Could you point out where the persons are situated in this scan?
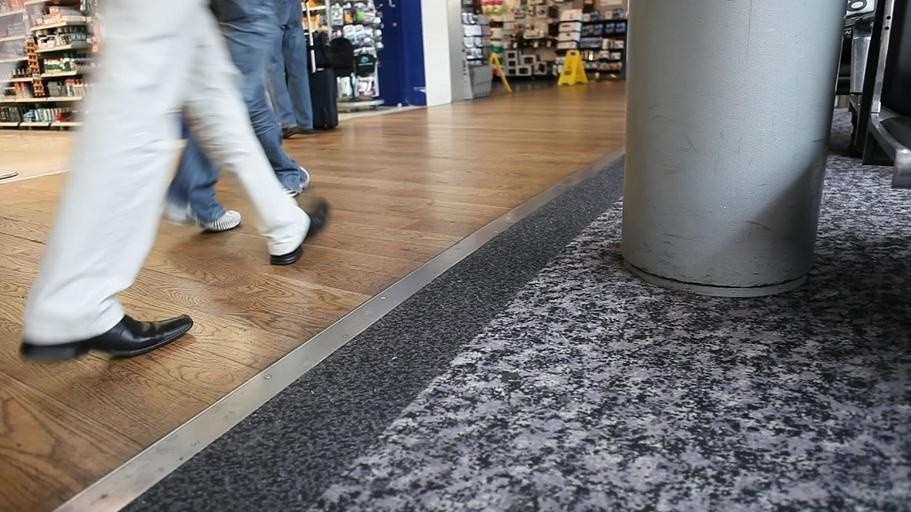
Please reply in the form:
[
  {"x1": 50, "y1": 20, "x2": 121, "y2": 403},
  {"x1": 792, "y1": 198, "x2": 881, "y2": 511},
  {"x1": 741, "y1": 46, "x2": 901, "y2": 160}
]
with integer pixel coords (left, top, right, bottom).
[
  {"x1": 164, "y1": 0, "x2": 312, "y2": 233},
  {"x1": 14, "y1": 0, "x2": 331, "y2": 363},
  {"x1": 266, "y1": 1, "x2": 319, "y2": 137}
]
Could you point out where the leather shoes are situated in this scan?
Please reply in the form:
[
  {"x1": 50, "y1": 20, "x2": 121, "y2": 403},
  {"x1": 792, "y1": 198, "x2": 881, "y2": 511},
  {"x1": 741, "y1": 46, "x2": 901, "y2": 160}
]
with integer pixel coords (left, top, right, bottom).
[
  {"x1": 17, "y1": 312, "x2": 196, "y2": 364},
  {"x1": 270, "y1": 193, "x2": 330, "y2": 267}
]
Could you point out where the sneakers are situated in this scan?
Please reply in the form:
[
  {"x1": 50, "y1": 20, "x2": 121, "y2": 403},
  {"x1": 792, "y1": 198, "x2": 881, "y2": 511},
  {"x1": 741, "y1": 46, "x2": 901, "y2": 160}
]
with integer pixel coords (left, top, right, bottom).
[
  {"x1": 161, "y1": 203, "x2": 198, "y2": 229},
  {"x1": 277, "y1": 126, "x2": 316, "y2": 140},
  {"x1": 197, "y1": 208, "x2": 245, "y2": 232},
  {"x1": 282, "y1": 165, "x2": 313, "y2": 199}
]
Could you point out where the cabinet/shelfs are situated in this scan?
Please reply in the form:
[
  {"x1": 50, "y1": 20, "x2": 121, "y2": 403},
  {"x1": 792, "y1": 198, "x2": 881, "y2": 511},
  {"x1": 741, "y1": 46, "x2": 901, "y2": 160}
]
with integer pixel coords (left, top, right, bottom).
[{"x1": 0, "y1": 0, "x2": 98, "y2": 129}]
